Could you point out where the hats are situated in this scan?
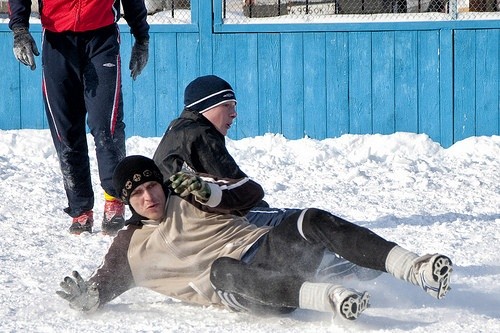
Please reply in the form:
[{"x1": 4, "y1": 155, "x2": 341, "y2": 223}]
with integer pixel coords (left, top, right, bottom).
[
  {"x1": 112, "y1": 155, "x2": 164, "y2": 205},
  {"x1": 183, "y1": 75, "x2": 237, "y2": 113}
]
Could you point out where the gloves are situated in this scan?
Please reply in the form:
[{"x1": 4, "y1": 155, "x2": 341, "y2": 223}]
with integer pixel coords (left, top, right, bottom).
[
  {"x1": 169, "y1": 171, "x2": 211, "y2": 203},
  {"x1": 129, "y1": 32, "x2": 150, "y2": 80},
  {"x1": 57, "y1": 271, "x2": 99, "y2": 311},
  {"x1": 13, "y1": 26, "x2": 40, "y2": 70}
]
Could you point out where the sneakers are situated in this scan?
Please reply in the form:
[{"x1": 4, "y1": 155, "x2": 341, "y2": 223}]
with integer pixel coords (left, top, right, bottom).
[
  {"x1": 101, "y1": 199, "x2": 125, "y2": 236},
  {"x1": 317, "y1": 255, "x2": 361, "y2": 283},
  {"x1": 406, "y1": 253, "x2": 453, "y2": 299},
  {"x1": 328, "y1": 287, "x2": 371, "y2": 321},
  {"x1": 69, "y1": 211, "x2": 94, "y2": 235}
]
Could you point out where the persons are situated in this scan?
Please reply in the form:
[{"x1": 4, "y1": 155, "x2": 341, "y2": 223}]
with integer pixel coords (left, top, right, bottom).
[
  {"x1": 151, "y1": 75, "x2": 383, "y2": 283},
  {"x1": 7, "y1": 0, "x2": 151, "y2": 234},
  {"x1": 56, "y1": 155, "x2": 452, "y2": 321}
]
